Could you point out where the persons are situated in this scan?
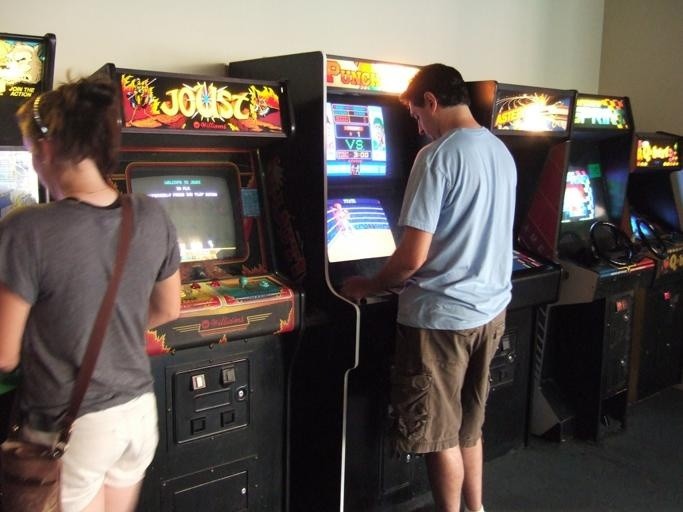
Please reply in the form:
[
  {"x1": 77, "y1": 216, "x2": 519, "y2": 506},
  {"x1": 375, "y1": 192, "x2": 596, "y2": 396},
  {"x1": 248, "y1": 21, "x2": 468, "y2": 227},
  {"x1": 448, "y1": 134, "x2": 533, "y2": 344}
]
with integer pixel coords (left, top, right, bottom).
[
  {"x1": 333, "y1": 200, "x2": 356, "y2": 236},
  {"x1": 333, "y1": 63, "x2": 519, "y2": 510},
  {"x1": 0, "y1": 66, "x2": 184, "y2": 512}
]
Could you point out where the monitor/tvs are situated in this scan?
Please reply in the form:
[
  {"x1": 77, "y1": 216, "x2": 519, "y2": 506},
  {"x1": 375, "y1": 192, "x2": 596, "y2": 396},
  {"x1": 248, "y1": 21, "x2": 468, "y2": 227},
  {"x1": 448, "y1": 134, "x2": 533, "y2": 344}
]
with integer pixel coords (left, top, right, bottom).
[
  {"x1": 327, "y1": 196, "x2": 397, "y2": 263},
  {"x1": 561, "y1": 169, "x2": 595, "y2": 223},
  {"x1": 130, "y1": 169, "x2": 241, "y2": 262},
  {"x1": 0, "y1": 150, "x2": 40, "y2": 219},
  {"x1": 326, "y1": 102, "x2": 387, "y2": 177}
]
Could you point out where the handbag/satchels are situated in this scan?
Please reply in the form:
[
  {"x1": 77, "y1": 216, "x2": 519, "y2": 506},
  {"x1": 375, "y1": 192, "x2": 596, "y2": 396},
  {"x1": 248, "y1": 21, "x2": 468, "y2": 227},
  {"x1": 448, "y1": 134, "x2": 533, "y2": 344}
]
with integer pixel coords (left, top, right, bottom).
[{"x1": 0, "y1": 435, "x2": 65, "y2": 512}]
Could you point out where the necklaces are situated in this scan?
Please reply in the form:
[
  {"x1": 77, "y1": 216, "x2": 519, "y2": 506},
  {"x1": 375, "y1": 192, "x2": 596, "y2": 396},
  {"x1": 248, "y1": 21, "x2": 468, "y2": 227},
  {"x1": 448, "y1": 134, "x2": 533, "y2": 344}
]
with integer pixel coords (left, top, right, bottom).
[{"x1": 58, "y1": 185, "x2": 111, "y2": 198}]
